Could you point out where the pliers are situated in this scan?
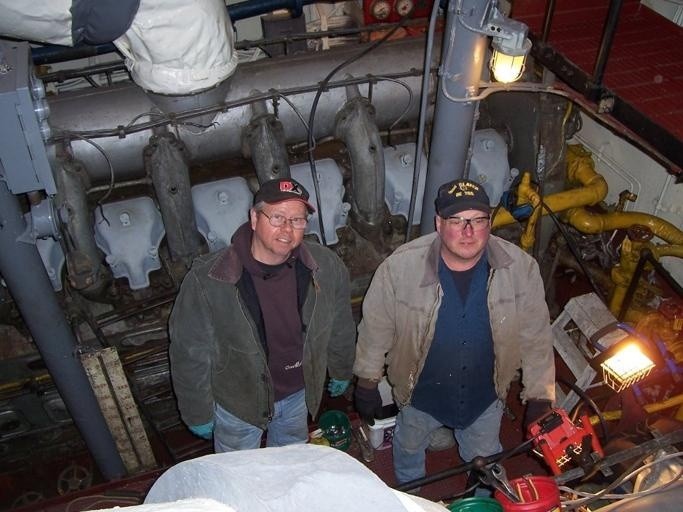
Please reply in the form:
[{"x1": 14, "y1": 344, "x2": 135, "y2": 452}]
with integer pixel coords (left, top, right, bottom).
[{"x1": 516, "y1": 479, "x2": 538, "y2": 503}]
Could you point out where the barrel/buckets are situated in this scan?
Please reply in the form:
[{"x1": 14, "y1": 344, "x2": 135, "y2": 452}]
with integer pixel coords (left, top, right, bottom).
[
  {"x1": 494, "y1": 475, "x2": 562, "y2": 511},
  {"x1": 446, "y1": 496, "x2": 502, "y2": 512},
  {"x1": 316, "y1": 410, "x2": 352, "y2": 452}
]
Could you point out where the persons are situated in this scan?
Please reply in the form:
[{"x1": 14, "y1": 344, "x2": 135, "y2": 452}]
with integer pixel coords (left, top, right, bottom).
[
  {"x1": 351, "y1": 179, "x2": 556, "y2": 498},
  {"x1": 167, "y1": 177, "x2": 356, "y2": 455}
]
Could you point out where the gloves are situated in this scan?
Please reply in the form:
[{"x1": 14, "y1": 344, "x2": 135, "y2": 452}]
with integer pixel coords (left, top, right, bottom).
[
  {"x1": 520, "y1": 397, "x2": 557, "y2": 461},
  {"x1": 327, "y1": 378, "x2": 352, "y2": 397},
  {"x1": 187, "y1": 417, "x2": 215, "y2": 441},
  {"x1": 351, "y1": 384, "x2": 384, "y2": 427}
]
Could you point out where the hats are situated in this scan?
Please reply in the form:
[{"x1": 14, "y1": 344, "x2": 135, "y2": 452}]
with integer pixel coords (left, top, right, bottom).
[
  {"x1": 252, "y1": 175, "x2": 317, "y2": 215},
  {"x1": 433, "y1": 178, "x2": 493, "y2": 219}
]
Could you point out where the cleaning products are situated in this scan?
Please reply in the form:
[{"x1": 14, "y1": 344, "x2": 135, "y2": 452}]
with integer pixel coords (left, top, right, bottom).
[{"x1": 309, "y1": 429, "x2": 329, "y2": 447}]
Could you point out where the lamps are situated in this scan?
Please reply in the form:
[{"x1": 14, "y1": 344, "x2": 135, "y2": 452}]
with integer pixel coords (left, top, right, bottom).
[
  {"x1": 599, "y1": 342, "x2": 657, "y2": 394},
  {"x1": 481, "y1": 10, "x2": 533, "y2": 85}
]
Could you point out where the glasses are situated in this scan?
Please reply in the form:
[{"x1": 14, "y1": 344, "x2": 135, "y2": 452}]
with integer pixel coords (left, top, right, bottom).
[
  {"x1": 439, "y1": 212, "x2": 492, "y2": 230},
  {"x1": 255, "y1": 206, "x2": 309, "y2": 232}
]
